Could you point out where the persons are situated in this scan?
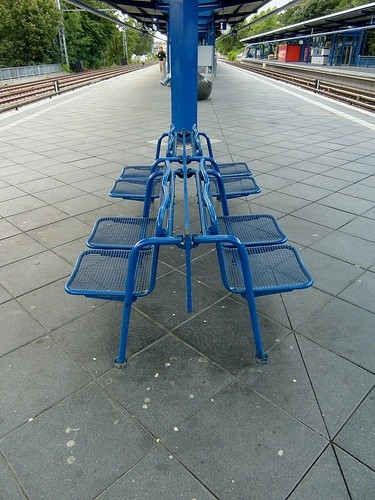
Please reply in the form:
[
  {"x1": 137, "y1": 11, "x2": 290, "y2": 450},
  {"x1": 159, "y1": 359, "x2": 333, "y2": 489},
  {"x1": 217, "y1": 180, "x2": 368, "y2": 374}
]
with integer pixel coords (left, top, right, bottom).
[{"x1": 157, "y1": 46, "x2": 166, "y2": 77}]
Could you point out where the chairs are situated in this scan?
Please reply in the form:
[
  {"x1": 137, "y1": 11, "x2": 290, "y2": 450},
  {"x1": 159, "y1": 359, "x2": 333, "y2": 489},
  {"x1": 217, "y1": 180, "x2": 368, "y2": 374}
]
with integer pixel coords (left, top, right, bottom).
[{"x1": 63, "y1": 121, "x2": 313, "y2": 368}]
